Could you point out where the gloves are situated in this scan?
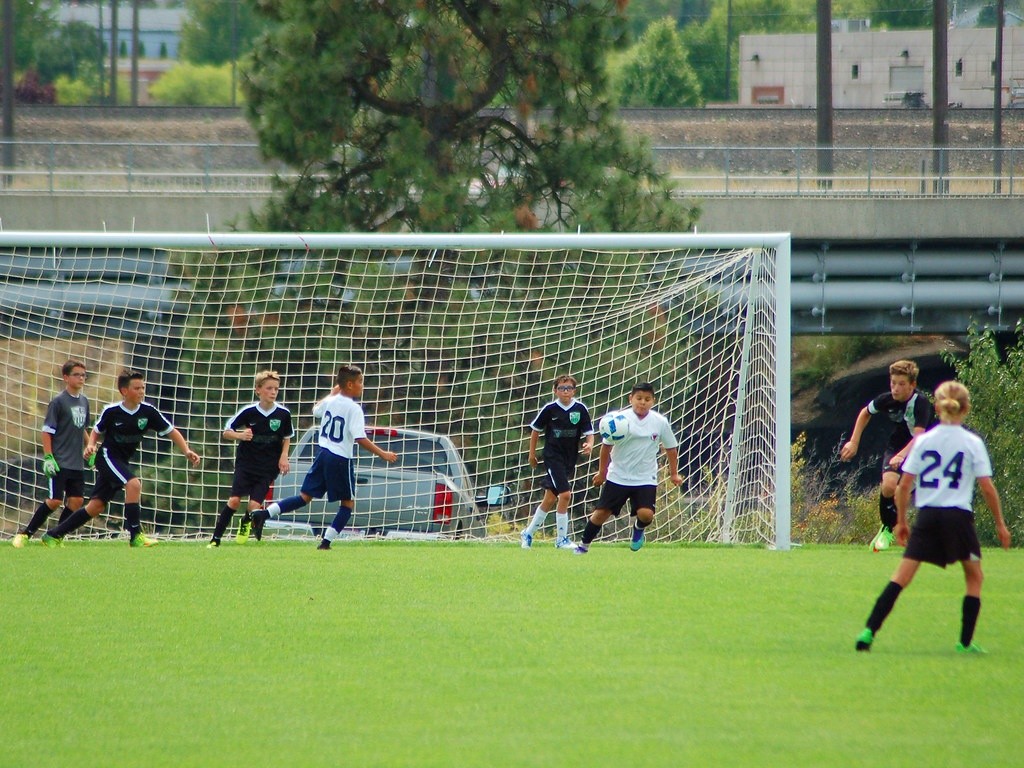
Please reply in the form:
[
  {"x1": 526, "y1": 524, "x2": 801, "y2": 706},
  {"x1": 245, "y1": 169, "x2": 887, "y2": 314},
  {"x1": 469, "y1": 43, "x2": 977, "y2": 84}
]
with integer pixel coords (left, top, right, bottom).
[
  {"x1": 88, "y1": 449, "x2": 98, "y2": 467},
  {"x1": 43, "y1": 453, "x2": 59, "y2": 478}
]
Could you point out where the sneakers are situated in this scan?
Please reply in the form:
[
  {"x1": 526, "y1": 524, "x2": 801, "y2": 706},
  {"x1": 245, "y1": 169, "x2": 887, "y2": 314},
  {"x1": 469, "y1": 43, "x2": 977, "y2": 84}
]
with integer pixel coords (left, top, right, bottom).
[
  {"x1": 630, "y1": 520, "x2": 646, "y2": 551},
  {"x1": 554, "y1": 536, "x2": 578, "y2": 552},
  {"x1": 856, "y1": 629, "x2": 873, "y2": 651},
  {"x1": 954, "y1": 638, "x2": 989, "y2": 657},
  {"x1": 869, "y1": 524, "x2": 896, "y2": 553},
  {"x1": 236, "y1": 517, "x2": 252, "y2": 545},
  {"x1": 129, "y1": 534, "x2": 157, "y2": 547},
  {"x1": 573, "y1": 540, "x2": 589, "y2": 555},
  {"x1": 251, "y1": 509, "x2": 264, "y2": 542},
  {"x1": 519, "y1": 528, "x2": 532, "y2": 549},
  {"x1": 40, "y1": 533, "x2": 65, "y2": 549},
  {"x1": 13, "y1": 534, "x2": 29, "y2": 549}
]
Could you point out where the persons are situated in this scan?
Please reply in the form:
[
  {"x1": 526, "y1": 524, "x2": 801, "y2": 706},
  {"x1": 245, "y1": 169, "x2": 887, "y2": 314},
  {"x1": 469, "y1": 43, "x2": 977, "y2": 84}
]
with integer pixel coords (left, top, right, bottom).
[
  {"x1": 520, "y1": 374, "x2": 594, "y2": 549},
  {"x1": 574, "y1": 381, "x2": 682, "y2": 554},
  {"x1": 13, "y1": 359, "x2": 98, "y2": 549},
  {"x1": 251, "y1": 365, "x2": 397, "y2": 550},
  {"x1": 207, "y1": 370, "x2": 295, "y2": 548},
  {"x1": 853, "y1": 383, "x2": 1012, "y2": 652},
  {"x1": 41, "y1": 369, "x2": 201, "y2": 547},
  {"x1": 839, "y1": 359, "x2": 940, "y2": 553}
]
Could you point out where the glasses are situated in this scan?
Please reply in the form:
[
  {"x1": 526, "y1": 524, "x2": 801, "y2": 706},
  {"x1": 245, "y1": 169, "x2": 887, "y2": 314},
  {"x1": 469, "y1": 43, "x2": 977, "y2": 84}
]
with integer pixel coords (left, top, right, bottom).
[
  {"x1": 558, "y1": 385, "x2": 574, "y2": 392},
  {"x1": 67, "y1": 372, "x2": 87, "y2": 379}
]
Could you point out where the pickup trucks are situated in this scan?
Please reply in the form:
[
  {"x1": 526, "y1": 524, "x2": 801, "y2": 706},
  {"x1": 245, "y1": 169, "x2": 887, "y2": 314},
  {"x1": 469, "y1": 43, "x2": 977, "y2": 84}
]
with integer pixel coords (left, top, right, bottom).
[{"x1": 248, "y1": 425, "x2": 511, "y2": 540}]
